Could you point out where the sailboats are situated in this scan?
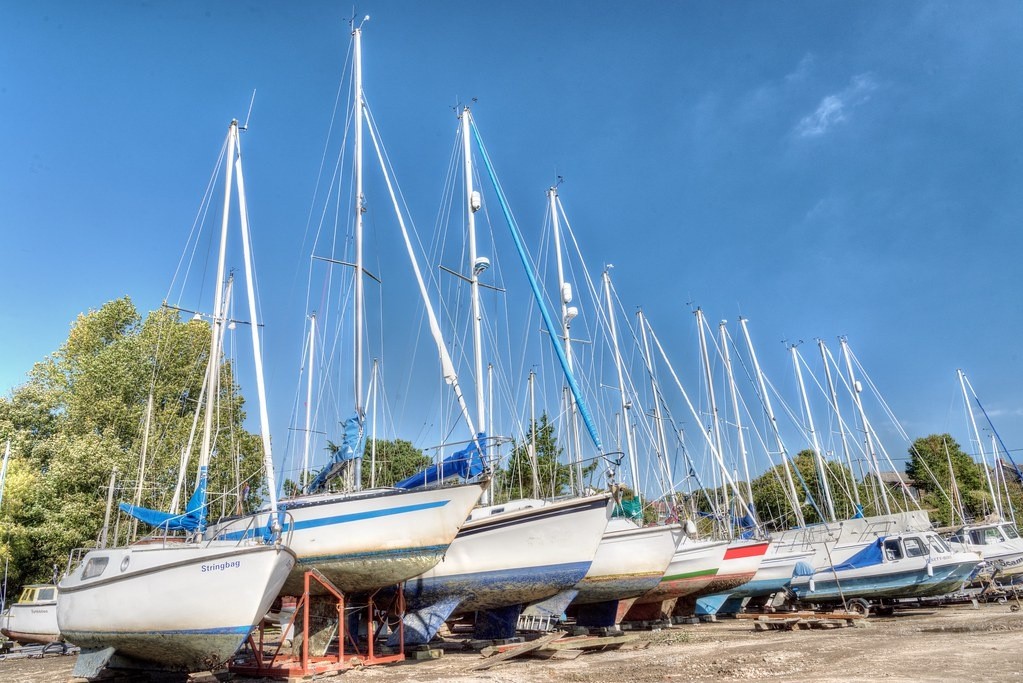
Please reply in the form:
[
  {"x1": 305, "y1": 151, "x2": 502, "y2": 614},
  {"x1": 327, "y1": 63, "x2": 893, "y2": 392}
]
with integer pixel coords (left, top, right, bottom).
[
  {"x1": 54, "y1": 116, "x2": 296, "y2": 676},
  {"x1": 379, "y1": 108, "x2": 1023, "y2": 639},
  {"x1": 200, "y1": 7, "x2": 493, "y2": 604}
]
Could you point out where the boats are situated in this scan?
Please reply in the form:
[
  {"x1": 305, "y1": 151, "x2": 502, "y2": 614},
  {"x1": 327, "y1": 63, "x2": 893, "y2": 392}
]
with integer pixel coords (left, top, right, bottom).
[{"x1": 0, "y1": 583, "x2": 66, "y2": 646}]
